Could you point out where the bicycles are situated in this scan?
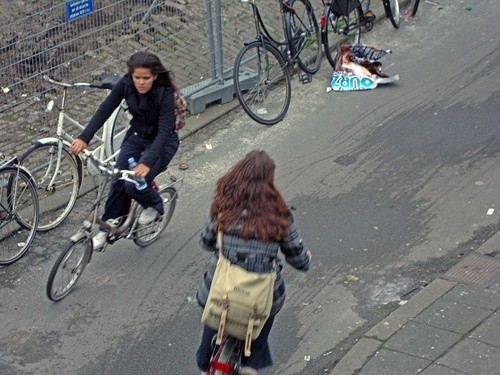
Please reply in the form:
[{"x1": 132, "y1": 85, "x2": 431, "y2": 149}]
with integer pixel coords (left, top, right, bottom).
[
  {"x1": 8, "y1": 73, "x2": 133, "y2": 233},
  {"x1": 233, "y1": 0, "x2": 323, "y2": 125},
  {"x1": 205, "y1": 206, "x2": 298, "y2": 375},
  {"x1": 411, "y1": 0, "x2": 420, "y2": 17},
  {"x1": 321, "y1": 0, "x2": 400, "y2": 70},
  {"x1": 0, "y1": 152, "x2": 40, "y2": 266},
  {"x1": 46, "y1": 146, "x2": 185, "y2": 301}
]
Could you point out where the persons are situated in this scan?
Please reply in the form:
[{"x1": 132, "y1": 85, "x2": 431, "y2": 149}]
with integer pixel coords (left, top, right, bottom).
[
  {"x1": 194, "y1": 149, "x2": 310, "y2": 374},
  {"x1": 68, "y1": 52, "x2": 181, "y2": 250}
]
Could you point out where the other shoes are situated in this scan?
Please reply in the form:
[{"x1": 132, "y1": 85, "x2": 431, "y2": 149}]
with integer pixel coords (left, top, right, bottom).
[{"x1": 241, "y1": 366, "x2": 259, "y2": 375}]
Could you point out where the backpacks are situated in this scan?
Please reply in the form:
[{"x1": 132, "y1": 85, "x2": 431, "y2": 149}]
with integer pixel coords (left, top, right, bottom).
[{"x1": 124, "y1": 88, "x2": 187, "y2": 130}]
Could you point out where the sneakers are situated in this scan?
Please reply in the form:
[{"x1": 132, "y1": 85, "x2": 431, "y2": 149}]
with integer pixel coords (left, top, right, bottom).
[
  {"x1": 92, "y1": 231, "x2": 108, "y2": 251},
  {"x1": 138, "y1": 207, "x2": 158, "y2": 225}
]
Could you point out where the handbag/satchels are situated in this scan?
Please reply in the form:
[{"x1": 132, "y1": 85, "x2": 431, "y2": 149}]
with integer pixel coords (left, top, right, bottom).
[{"x1": 199, "y1": 254, "x2": 278, "y2": 357}]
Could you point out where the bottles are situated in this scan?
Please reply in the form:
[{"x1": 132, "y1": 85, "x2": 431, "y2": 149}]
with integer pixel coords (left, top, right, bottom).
[{"x1": 128, "y1": 157, "x2": 147, "y2": 190}]
